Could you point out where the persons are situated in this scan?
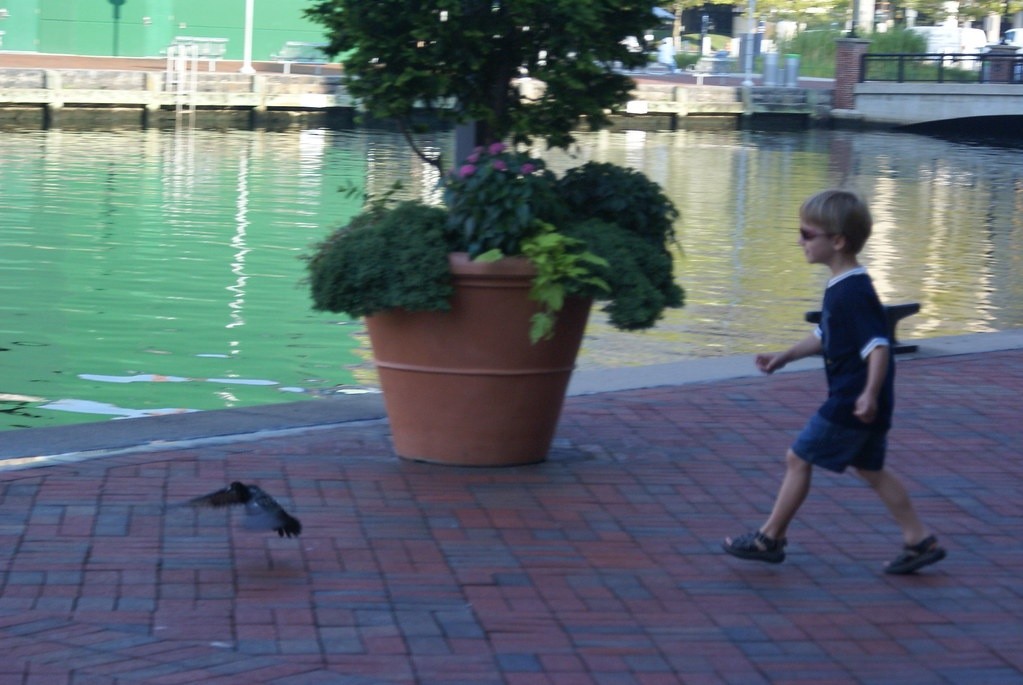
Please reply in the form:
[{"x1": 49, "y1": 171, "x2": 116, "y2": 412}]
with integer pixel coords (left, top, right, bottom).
[{"x1": 718, "y1": 187, "x2": 948, "y2": 576}]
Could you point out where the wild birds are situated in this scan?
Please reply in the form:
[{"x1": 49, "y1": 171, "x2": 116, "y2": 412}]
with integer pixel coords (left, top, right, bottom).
[{"x1": 180, "y1": 481, "x2": 302, "y2": 540}]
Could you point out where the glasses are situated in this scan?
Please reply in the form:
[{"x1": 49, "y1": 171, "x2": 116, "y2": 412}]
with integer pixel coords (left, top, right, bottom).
[{"x1": 800, "y1": 228, "x2": 834, "y2": 240}]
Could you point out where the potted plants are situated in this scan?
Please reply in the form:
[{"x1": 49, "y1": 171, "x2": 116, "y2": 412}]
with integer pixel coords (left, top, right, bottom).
[{"x1": 296, "y1": 1, "x2": 682, "y2": 469}]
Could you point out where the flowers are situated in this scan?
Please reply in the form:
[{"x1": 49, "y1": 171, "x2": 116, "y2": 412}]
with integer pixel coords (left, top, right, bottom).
[{"x1": 438, "y1": 142, "x2": 554, "y2": 250}]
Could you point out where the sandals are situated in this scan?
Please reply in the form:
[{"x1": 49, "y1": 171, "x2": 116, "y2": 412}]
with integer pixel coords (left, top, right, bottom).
[
  {"x1": 884, "y1": 534, "x2": 945, "y2": 574},
  {"x1": 722, "y1": 530, "x2": 787, "y2": 563}
]
